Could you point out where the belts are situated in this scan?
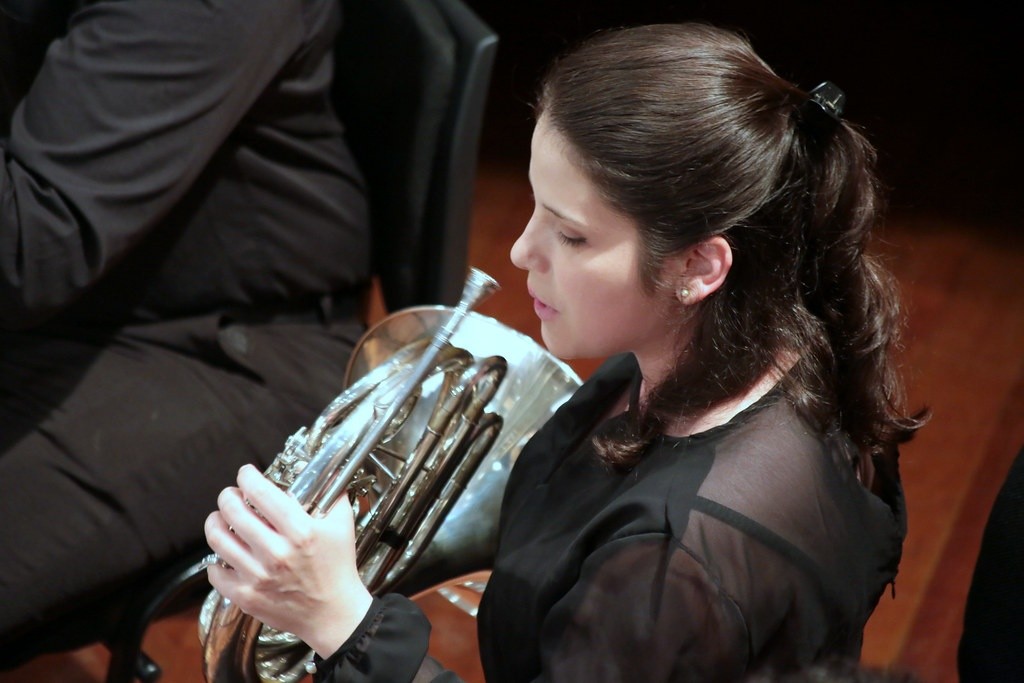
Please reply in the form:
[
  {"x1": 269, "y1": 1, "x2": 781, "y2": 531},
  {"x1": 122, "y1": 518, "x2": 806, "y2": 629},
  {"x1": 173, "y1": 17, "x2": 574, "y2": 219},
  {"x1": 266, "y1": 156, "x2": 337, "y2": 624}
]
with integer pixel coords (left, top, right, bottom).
[{"x1": 223, "y1": 283, "x2": 364, "y2": 318}]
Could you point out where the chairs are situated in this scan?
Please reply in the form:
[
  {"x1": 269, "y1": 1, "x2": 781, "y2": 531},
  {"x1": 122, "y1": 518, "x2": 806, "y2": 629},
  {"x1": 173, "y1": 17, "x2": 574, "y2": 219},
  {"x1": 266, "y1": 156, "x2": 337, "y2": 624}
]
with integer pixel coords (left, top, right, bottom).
[{"x1": 0, "y1": 0, "x2": 498, "y2": 683}]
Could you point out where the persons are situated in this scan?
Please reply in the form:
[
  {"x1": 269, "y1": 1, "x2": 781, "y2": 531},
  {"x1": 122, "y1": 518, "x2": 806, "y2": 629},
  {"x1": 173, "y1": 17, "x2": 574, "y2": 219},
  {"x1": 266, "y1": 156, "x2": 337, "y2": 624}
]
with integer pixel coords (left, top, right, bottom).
[
  {"x1": 0, "y1": 0, "x2": 373, "y2": 643},
  {"x1": 203, "y1": 23, "x2": 930, "y2": 683}
]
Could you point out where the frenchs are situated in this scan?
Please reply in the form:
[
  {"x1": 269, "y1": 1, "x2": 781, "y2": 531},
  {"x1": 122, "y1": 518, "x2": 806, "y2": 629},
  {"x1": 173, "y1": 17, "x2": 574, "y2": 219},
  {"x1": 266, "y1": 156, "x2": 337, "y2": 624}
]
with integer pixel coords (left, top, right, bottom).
[{"x1": 198, "y1": 263, "x2": 586, "y2": 683}]
